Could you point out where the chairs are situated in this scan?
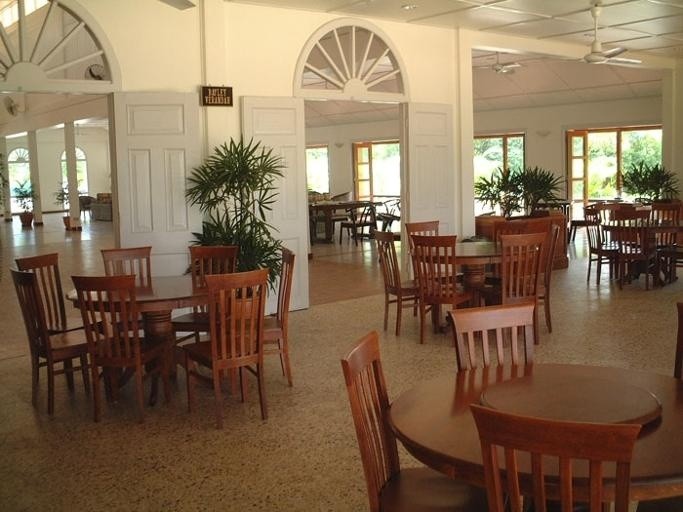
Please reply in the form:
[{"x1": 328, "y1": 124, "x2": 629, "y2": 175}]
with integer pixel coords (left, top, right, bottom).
[
  {"x1": 9, "y1": 269, "x2": 106, "y2": 417},
  {"x1": 81, "y1": 192, "x2": 99, "y2": 213},
  {"x1": 263, "y1": 241, "x2": 297, "y2": 390},
  {"x1": 370, "y1": 218, "x2": 559, "y2": 340},
  {"x1": 171, "y1": 244, "x2": 238, "y2": 344},
  {"x1": 183, "y1": 268, "x2": 268, "y2": 431},
  {"x1": 341, "y1": 333, "x2": 482, "y2": 512},
  {"x1": 446, "y1": 299, "x2": 539, "y2": 368},
  {"x1": 99, "y1": 245, "x2": 153, "y2": 330},
  {"x1": 309, "y1": 184, "x2": 407, "y2": 247},
  {"x1": 468, "y1": 400, "x2": 646, "y2": 510},
  {"x1": 583, "y1": 198, "x2": 683, "y2": 288},
  {"x1": 73, "y1": 275, "x2": 174, "y2": 425},
  {"x1": 14, "y1": 251, "x2": 108, "y2": 334}
]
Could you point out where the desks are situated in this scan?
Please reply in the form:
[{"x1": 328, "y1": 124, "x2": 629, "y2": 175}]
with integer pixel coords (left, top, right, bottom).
[
  {"x1": 66, "y1": 274, "x2": 233, "y2": 402},
  {"x1": 388, "y1": 362, "x2": 681, "y2": 500}
]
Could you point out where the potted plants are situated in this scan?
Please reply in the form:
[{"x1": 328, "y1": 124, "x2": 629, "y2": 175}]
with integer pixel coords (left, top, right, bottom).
[
  {"x1": 56, "y1": 190, "x2": 71, "y2": 229},
  {"x1": 12, "y1": 182, "x2": 36, "y2": 227}
]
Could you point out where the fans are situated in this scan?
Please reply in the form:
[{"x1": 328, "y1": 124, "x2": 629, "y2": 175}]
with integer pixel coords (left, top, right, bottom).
[
  {"x1": 569, "y1": 6, "x2": 646, "y2": 69},
  {"x1": 471, "y1": 50, "x2": 530, "y2": 78}
]
